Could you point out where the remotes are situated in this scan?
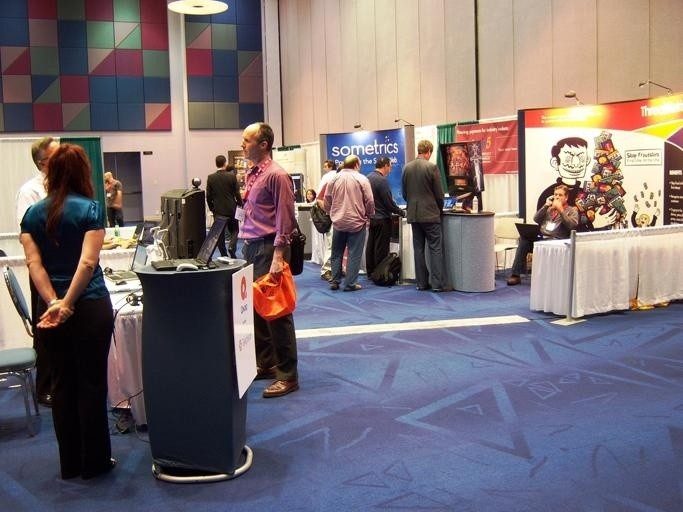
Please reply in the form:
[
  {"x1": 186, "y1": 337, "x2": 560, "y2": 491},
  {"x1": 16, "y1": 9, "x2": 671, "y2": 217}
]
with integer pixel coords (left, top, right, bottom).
[{"x1": 216, "y1": 256, "x2": 230, "y2": 263}]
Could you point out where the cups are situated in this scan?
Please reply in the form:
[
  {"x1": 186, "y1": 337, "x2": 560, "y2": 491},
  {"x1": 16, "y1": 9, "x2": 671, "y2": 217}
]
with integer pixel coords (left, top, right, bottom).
[{"x1": 455, "y1": 201, "x2": 463, "y2": 211}]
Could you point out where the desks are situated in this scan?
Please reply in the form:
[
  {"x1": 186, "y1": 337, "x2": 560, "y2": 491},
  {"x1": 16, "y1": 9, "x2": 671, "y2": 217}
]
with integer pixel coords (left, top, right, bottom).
[
  {"x1": 98, "y1": 242, "x2": 159, "y2": 434},
  {"x1": 438, "y1": 211, "x2": 495, "y2": 293},
  {"x1": 529, "y1": 225, "x2": 683, "y2": 317},
  {"x1": 309, "y1": 218, "x2": 416, "y2": 280},
  {"x1": 292, "y1": 206, "x2": 312, "y2": 258}
]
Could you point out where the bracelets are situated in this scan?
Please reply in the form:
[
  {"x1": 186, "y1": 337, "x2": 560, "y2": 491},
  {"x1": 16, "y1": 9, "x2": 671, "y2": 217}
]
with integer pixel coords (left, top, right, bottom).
[{"x1": 45, "y1": 294, "x2": 61, "y2": 307}]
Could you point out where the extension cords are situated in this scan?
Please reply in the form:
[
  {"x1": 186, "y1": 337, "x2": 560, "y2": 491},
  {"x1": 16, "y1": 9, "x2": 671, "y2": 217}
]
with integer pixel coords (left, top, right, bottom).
[{"x1": 116, "y1": 420, "x2": 130, "y2": 433}]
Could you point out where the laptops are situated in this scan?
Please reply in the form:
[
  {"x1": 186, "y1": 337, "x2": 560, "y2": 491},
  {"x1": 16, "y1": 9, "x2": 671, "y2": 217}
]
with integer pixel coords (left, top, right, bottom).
[
  {"x1": 104, "y1": 244, "x2": 149, "y2": 282},
  {"x1": 442, "y1": 197, "x2": 457, "y2": 212},
  {"x1": 151, "y1": 214, "x2": 230, "y2": 271},
  {"x1": 515, "y1": 222, "x2": 538, "y2": 242}
]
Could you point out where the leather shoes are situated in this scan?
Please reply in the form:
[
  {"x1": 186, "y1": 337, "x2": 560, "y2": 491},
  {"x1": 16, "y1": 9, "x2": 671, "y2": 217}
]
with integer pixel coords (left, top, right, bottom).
[
  {"x1": 82, "y1": 458, "x2": 117, "y2": 481},
  {"x1": 36, "y1": 392, "x2": 52, "y2": 406},
  {"x1": 263, "y1": 379, "x2": 299, "y2": 398},
  {"x1": 254, "y1": 365, "x2": 276, "y2": 380},
  {"x1": 418, "y1": 285, "x2": 431, "y2": 290},
  {"x1": 228, "y1": 247, "x2": 236, "y2": 258},
  {"x1": 433, "y1": 286, "x2": 453, "y2": 293}
]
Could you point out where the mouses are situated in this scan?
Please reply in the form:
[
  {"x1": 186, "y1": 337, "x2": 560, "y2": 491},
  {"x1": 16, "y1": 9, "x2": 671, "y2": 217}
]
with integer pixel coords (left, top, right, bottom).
[
  {"x1": 176, "y1": 262, "x2": 197, "y2": 272},
  {"x1": 115, "y1": 279, "x2": 126, "y2": 286}
]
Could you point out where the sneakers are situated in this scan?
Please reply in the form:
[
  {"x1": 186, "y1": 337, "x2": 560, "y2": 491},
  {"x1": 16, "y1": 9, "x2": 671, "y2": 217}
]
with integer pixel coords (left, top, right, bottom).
[
  {"x1": 321, "y1": 271, "x2": 361, "y2": 292},
  {"x1": 507, "y1": 276, "x2": 521, "y2": 285}
]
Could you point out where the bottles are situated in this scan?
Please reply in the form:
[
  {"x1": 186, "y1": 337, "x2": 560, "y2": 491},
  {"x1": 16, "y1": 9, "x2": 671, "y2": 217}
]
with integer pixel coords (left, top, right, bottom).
[
  {"x1": 114, "y1": 225, "x2": 120, "y2": 237},
  {"x1": 472, "y1": 196, "x2": 478, "y2": 214}
]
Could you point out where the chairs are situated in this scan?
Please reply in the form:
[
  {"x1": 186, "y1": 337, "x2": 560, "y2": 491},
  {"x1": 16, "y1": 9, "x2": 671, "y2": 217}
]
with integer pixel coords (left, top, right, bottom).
[
  {"x1": 3, "y1": 264, "x2": 35, "y2": 337},
  {"x1": 494, "y1": 218, "x2": 523, "y2": 276},
  {"x1": 0, "y1": 348, "x2": 37, "y2": 436}
]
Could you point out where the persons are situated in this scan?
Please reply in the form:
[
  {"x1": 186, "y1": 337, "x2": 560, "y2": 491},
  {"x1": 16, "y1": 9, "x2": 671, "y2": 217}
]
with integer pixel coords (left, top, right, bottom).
[
  {"x1": 365, "y1": 156, "x2": 408, "y2": 278},
  {"x1": 238, "y1": 122, "x2": 304, "y2": 397},
  {"x1": 302, "y1": 187, "x2": 319, "y2": 205},
  {"x1": 317, "y1": 161, "x2": 347, "y2": 281},
  {"x1": 224, "y1": 163, "x2": 238, "y2": 245},
  {"x1": 309, "y1": 159, "x2": 339, "y2": 264},
  {"x1": 20, "y1": 143, "x2": 117, "y2": 482},
  {"x1": 506, "y1": 184, "x2": 579, "y2": 285},
  {"x1": 323, "y1": 154, "x2": 376, "y2": 290},
  {"x1": 14, "y1": 135, "x2": 63, "y2": 407},
  {"x1": 401, "y1": 140, "x2": 454, "y2": 292},
  {"x1": 103, "y1": 170, "x2": 125, "y2": 226},
  {"x1": 448, "y1": 176, "x2": 477, "y2": 211},
  {"x1": 205, "y1": 155, "x2": 246, "y2": 258}
]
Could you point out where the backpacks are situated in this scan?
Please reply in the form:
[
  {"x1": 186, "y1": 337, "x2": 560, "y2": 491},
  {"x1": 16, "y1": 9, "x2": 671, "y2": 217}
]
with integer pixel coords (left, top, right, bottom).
[{"x1": 371, "y1": 253, "x2": 401, "y2": 286}]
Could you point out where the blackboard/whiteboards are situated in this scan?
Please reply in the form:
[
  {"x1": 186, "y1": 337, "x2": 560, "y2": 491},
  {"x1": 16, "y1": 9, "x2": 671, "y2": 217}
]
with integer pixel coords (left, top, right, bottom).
[{"x1": 0, "y1": 136, "x2": 60, "y2": 240}]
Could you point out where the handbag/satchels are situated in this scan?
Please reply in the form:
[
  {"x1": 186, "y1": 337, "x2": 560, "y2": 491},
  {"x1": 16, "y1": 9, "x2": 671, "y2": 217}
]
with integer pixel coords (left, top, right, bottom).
[
  {"x1": 310, "y1": 201, "x2": 332, "y2": 233},
  {"x1": 254, "y1": 261, "x2": 297, "y2": 322},
  {"x1": 390, "y1": 215, "x2": 399, "y2": 239},
  {"x1": 290, "y1": 232, "x2": 306, "y2": 275}
]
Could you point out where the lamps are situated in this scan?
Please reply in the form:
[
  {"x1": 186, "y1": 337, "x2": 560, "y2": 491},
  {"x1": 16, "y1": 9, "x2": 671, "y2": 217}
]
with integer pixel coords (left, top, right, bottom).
[
  {"x1": 638, "y1": 80, "x2": 673, "y2": 96},
  {"x1": 167, "y1": 0, "x2": 229, "y2": 17},
  {"x1": 564, "y1": 91, "x2": 586, "y2": 107}
]
